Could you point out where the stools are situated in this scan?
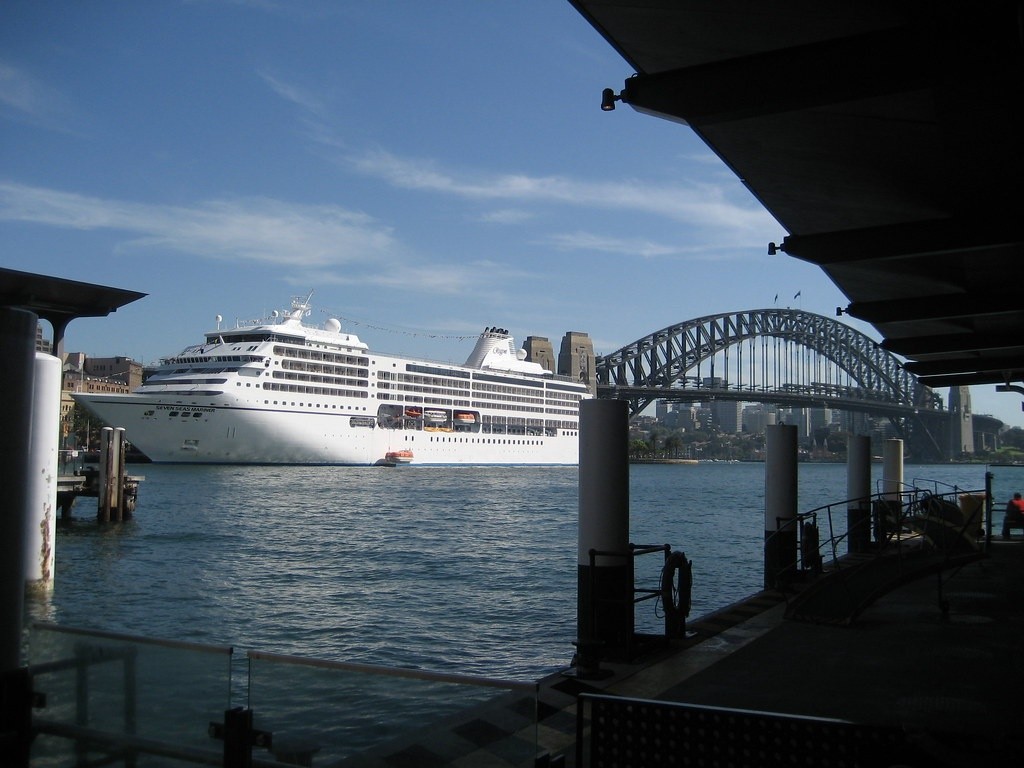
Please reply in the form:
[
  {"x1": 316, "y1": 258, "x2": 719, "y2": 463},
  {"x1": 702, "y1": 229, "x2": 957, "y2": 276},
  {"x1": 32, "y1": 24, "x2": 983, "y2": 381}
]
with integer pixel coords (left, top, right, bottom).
[{"x1": 1003, "y1": 519, "x2": 1024, "y2": 539}]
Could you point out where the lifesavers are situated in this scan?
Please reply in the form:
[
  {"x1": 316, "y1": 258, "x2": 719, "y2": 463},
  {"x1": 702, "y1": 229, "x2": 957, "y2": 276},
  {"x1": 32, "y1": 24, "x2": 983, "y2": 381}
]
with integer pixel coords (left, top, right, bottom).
[{"x1": 662, "y1": 551, "x2": 693, "y2": 616}]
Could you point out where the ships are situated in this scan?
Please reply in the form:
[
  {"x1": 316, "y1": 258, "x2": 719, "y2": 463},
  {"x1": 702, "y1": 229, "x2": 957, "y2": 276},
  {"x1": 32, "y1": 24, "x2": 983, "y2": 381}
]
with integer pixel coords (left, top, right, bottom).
[{"x1": 67, "y1": 291, "x2": 594, "y2": 466}]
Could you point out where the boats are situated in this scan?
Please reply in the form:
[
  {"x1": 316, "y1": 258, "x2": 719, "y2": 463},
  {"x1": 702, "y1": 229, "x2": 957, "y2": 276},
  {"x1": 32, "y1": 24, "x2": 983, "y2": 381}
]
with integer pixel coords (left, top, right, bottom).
[
  {"x1": 384, "y1": 451, "x2": 415, "y2": 463},
  {"x1": 424, "y1": 426, "x2": 437, "y2": 432},
  {"x1": 405, "y1": 408, "x2": 421, "y2": 417},
  {"x1": 424, "y1": 410, "x2": 449, "y2": 422},
  {"x1": 439, "y1": 427, "x2": 452, "y2": 433},
  {"x1": 456, "y1": 414, "x2": 476, "y2": 425}
]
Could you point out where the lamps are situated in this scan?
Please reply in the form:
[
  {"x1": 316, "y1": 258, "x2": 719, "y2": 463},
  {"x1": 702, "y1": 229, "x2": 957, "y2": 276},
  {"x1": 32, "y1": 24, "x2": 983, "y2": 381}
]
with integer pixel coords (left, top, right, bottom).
[
  {"x1": 601, "y1": 89, "x2": 627, "y2": 111},
  {"x1": 768, "y1": 243, "x2": 784, "y2": 255},
  {"x1": 836, "y1": 307, "x2": 848, "y2": 316}
]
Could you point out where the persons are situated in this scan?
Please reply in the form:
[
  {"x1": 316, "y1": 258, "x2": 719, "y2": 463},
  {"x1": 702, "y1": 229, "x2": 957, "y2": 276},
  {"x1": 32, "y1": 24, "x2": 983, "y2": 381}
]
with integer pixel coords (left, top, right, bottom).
[{"x1": 1002, "y1": 492, "x2": 1024, "y2": 541}]
